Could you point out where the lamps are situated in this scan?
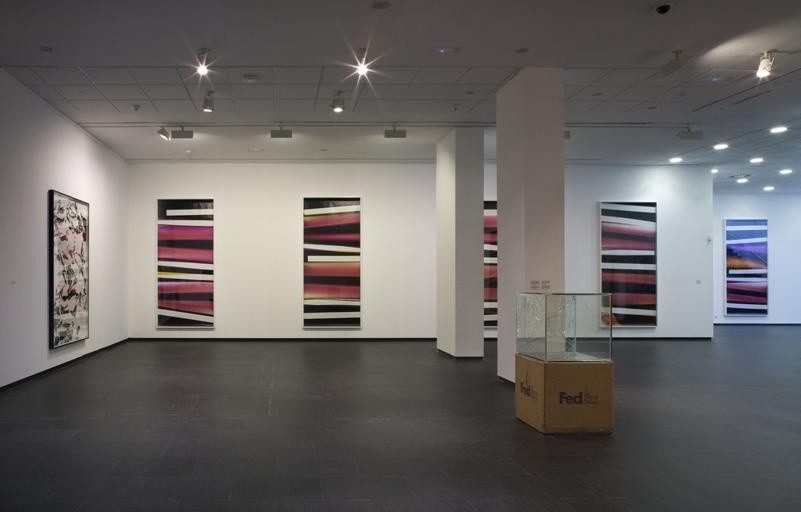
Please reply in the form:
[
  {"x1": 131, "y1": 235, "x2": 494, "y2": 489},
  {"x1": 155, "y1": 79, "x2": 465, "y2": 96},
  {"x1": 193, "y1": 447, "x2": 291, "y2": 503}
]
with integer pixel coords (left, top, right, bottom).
[
  {"x1": 158, "y1": 47, "x2": 406, "y2": 142},
  {"x1": 756, "y1": 48, "x2": 801, "y2": 78}
]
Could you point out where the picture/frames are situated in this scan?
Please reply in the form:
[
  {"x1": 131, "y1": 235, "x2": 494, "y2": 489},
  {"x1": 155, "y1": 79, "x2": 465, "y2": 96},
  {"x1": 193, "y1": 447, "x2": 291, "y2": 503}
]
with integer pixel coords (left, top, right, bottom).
[{"x1": 48, "y1": 189, "x2": 89, "y2": 349}]
